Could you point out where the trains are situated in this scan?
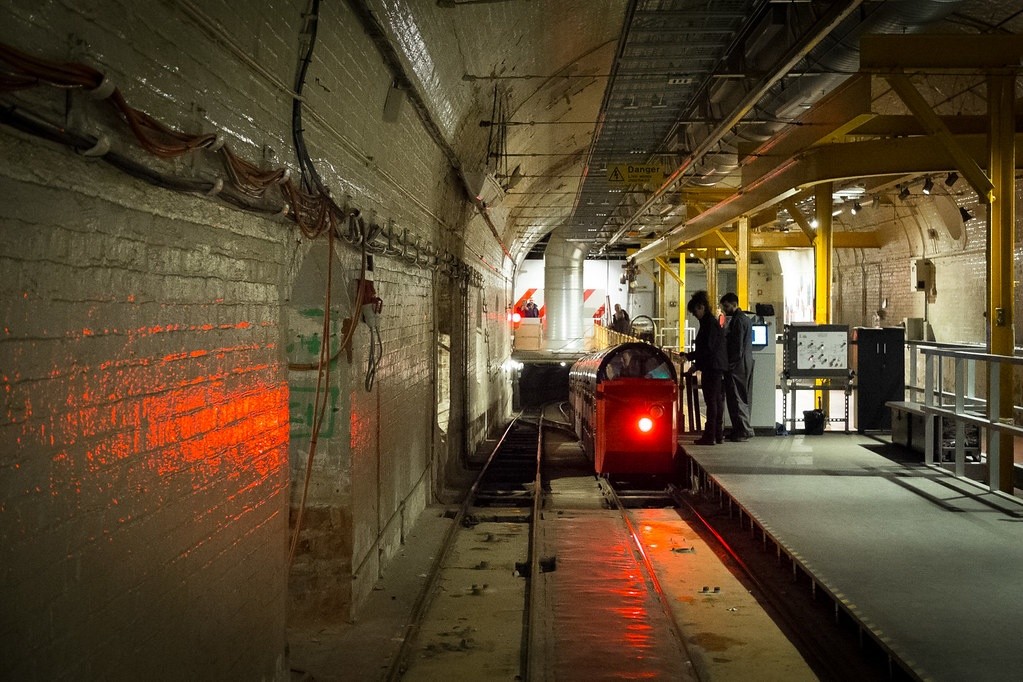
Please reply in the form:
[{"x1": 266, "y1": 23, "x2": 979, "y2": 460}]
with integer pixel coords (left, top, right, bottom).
[{"x1": 568, "y1": 341, "x2": 681, "y2": 486}]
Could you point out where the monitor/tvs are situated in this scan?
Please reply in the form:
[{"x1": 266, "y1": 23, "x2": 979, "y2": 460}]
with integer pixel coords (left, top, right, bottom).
[{"x1": 751, "y1": 324, "x2": 768, "y2": 346}]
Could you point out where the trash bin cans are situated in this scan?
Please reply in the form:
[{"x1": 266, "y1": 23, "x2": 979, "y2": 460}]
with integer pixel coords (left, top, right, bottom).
[{"x1": 803, "y1": 409, "x2": 826, "y2": 435}]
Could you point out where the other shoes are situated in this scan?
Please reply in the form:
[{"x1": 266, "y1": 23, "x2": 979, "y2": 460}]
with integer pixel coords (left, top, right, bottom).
[
  {"x1": 715, "y1": 439, "x2": 724, "y2": 444},
  {"x1": 694, "y1": 439, "x2": 715, "y2": 445},
  {"x1": 725, "y1": 430, "x2": 755, "y2": 442}
]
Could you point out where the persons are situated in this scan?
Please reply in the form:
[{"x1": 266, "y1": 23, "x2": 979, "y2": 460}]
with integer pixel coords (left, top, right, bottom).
[
  {"x1": 677, "y1": 289, "x2": 724, "y2": 446},
  {"x1": 613, "y1": 304, "x2": 631, "y2": 336},
  {"x1": 519, "y1": 297, "x2": 539, "y2": 318},
  {"x1": 720, "y1": 292, "x2": 756, "y2": 442}
]
